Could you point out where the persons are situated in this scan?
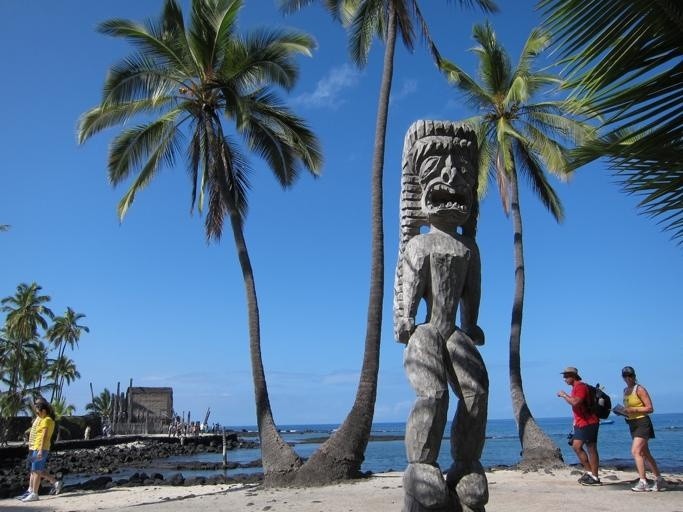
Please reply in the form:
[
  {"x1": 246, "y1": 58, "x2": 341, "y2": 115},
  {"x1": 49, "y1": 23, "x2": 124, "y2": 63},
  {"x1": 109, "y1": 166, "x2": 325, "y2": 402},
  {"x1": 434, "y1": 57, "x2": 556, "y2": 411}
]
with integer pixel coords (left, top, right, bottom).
[
  {"x1": 556, "y1": 367, "x2": 611, "y2": 485},
  {"x1": 16, "y1": 397, "x2": 55, "y2": 500},
  {"x1": 20, "y1": 402, "x2": 64, "y2": 503},
  {"x1": 607, "y1": 366, "x2": 667, "y2": 492}
]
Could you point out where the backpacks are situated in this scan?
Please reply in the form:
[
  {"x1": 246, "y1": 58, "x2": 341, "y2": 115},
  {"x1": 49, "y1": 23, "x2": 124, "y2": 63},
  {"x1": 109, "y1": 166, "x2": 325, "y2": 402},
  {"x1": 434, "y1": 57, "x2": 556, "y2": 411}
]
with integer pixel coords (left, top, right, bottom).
[{"x1": 573, "y1": 382, "x2": 612, "y2": 419}]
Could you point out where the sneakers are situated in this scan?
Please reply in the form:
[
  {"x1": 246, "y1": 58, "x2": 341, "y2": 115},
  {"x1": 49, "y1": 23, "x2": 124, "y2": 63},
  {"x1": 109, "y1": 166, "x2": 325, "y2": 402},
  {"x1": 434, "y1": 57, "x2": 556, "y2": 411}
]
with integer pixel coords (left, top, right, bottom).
[
  {"x1": 649, "y1": 478, "x2": 668, "y2": 491},
  {"x1": 584, "y1": 476, "x2": 602, "y2": 486},
  {"x1": 631, "y1": 481, "x2": 650, "y2": 492},
  {"x1": 17, "y1": 479, "x2": 64, "y2": 502},
  {"x1": 578, "y1": 474, "x2": 591, "y2": 484}
]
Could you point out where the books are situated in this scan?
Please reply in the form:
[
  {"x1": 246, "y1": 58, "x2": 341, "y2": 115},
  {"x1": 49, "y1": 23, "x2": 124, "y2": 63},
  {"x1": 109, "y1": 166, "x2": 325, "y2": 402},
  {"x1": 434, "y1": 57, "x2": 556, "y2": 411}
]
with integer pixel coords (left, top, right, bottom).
[{"x1": 608, "y1": 403, "x2": 632, "y2": 418}]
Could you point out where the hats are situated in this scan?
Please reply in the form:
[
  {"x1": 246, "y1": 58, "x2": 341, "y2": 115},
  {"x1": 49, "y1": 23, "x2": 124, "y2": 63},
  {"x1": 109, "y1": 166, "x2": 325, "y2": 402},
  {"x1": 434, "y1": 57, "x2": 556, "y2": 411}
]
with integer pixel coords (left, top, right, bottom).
[
  {"x1": 622, "y1": 366, "x2": 634, "y2": 377},
  {"x1": 559, "y1": 367, "x2": 581, "y2": 381}
]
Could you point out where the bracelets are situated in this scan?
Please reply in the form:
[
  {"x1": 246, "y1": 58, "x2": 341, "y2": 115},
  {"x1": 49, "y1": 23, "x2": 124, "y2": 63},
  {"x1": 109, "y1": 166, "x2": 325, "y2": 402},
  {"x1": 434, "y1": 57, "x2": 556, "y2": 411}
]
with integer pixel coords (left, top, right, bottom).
[{"x1": 563, "y1": 394, "x2": 567, "y2": 398}]
